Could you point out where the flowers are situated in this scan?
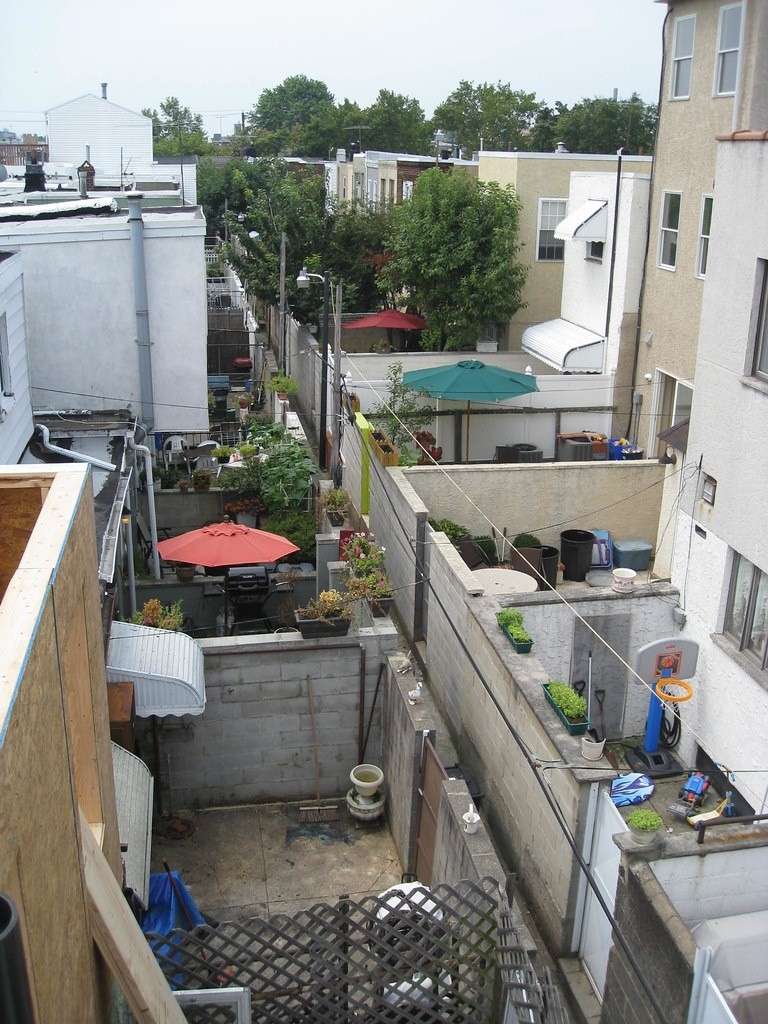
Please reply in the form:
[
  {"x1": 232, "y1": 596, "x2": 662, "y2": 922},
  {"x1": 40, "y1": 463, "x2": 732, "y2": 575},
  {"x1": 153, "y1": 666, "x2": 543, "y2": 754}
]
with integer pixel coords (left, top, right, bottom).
[
  {"x1": 223, "y1": 494, "x2": 268, "y2": 517},
  {"x1": 340, "y1": 532, "x2": 393, "y2": 599},
  {"x1": 124, "y1": 597, "x2": 186, "y2": 631},
  {"x1": 167, "y1": 560, "x2": 196, "y2": 568}
]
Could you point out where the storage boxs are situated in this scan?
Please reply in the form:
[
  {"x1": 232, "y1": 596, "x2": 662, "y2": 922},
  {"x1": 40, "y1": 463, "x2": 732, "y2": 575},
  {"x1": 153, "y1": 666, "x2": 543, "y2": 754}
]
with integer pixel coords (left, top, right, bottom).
[{"x1": 612, "y1": 539, "x2": 653, "y2": 571}]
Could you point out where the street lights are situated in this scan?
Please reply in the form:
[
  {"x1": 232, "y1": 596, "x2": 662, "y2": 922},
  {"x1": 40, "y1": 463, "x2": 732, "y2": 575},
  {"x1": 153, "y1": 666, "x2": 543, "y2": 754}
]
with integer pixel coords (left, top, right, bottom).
[
  {"x1": 296, "y1": 266, "x2": 330, "y2": 472},
  {"x1": 238, "y1": 213, "x2": 250, "y2": 303},
  {"x1": 248, "y1": 230, "x2": 286, "y2": 380}
]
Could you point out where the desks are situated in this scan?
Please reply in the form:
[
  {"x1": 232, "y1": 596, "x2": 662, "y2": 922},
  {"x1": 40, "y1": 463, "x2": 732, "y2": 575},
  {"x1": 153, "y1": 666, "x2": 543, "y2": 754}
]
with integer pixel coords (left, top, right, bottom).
[
  {"x1": 203, "y1": 581, "x2": 294, "y2": 607},
  {"x1": 472, "y1": 568, "x2": 539, "y2": 595}
]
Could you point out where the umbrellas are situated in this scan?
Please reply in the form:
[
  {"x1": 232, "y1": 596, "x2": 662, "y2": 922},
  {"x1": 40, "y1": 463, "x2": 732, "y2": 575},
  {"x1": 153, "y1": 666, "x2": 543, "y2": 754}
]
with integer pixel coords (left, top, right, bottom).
[
  {"x1": 156, "y1": 515, "x2": 300, "y2": 637},
  {"x1": 403, "y1": 358, "x2": 540, "y2": 465},
  {"x1": 342, "y1": 309, "x2": 426, "y2": 352}
]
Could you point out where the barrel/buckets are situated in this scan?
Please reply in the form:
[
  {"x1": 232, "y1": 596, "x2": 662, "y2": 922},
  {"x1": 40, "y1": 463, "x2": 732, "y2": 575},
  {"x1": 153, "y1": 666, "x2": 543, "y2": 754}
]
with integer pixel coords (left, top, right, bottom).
[
  {"x1": 529, "y1": 545, "x2": 559, "y2": 591},
  {"x1": 560, "y1": 529, "x2": 595, "y2": 582},
  {"x1": 612, "y1": 568, "x2": 637, "y2": 593}
]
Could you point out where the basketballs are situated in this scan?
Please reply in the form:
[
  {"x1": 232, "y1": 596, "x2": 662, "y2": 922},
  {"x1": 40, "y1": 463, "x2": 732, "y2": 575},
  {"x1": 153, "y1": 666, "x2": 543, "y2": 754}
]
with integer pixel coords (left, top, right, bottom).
[{"x1": 662, "y1": 656, "x2": 674, "y2": 667}]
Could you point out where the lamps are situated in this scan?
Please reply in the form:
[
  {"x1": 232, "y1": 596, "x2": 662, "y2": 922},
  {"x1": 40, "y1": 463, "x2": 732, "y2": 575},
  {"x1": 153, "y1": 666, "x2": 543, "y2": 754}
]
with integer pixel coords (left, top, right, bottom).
[{"x1": 658, "y1": 446, "x2": 677, "y2": 465}]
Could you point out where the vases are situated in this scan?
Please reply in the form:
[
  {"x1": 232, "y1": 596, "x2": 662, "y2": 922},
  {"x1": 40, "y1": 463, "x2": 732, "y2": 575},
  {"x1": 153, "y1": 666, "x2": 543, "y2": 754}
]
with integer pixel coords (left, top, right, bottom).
[
  {"x1": 346, "y1": 786, "x2": 387, "y2": 829},
  {"x1": 476, "y1": 340, "x2": 498, "y2": 353},
  {"x1": 274, "y1": 627, "x2": 299, "y2": 634},
  {"x1": 236, "y1": 512, "x2": 256, "y2": 528},
  {"x1": 373, "y1": 440, "x2": 400, "y2": 468},
  {"x1": 176, "y1": 566, "x2": 194, "y2": 583},
  {"x1": 349, "y1": 764, "x2": 384, "y2": 796},
  {"x1": 612, "y1": 567, "x2": 636, "y2": 594},
  {"x1": 351, "y1": 561, "x2": 379, "y2": 578},
  {"x1": 580, "y1": 735, "x2": 606, "y2": 762},
  {"x1": 367, "y1": 429, "x2": 390, "y2": 449},
  {"x1": 343, "y1": 392, "x2": 361, "y2": 413},
  {"x1": 366, "y1": 596, "x2": 395, "y2": 617}
]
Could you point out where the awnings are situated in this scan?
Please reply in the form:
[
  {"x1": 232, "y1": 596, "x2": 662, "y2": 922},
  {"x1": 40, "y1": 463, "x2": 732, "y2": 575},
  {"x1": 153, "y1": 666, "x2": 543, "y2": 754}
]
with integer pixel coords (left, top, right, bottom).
[
  {"x1": 522, "y1": 317, "x2": 604, "y2": 373},
  {"x1": 106, "y1": 621, "x2": 206, "y2": 717},
  {"x1": 554, "y1": 199, "x2": 608, "y2": 243},
  {"x1": 110, "y1": 741, "x2": 154, "y2": 911}
]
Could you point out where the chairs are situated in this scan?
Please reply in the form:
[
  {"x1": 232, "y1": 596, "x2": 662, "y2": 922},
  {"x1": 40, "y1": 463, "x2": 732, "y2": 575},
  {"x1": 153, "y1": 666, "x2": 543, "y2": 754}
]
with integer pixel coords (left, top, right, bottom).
[
  {"x1": 458, "y1": 536, "x2": 494, "y2": 571},
  {"x1": 376, "y1": 909, "x2": 439, "y2": 1012},
  {"x1": 216, "y1": 450, "x2": 241, "y2": 479},
  {"x1": 492, "y1": 446, "x2": 521, "y2": 463},
  {"x1": 226, "y1": 586, "x2": 274, "y2": 637},
  {"x1": 192, "y1": 440, "x2": 220, "y2": 474},
  {"x1": 135, "y1": 511, "x2": 172, "y2": 559},
  {"x1": 510, "y1": 548, "x2": 547, "y2": 591},
  {"x1": 163, "y1": 435, "x2": 191, "y2": 475},
  {"x1": 590, "y1": 529, "x2": 614, "y2": 574}
]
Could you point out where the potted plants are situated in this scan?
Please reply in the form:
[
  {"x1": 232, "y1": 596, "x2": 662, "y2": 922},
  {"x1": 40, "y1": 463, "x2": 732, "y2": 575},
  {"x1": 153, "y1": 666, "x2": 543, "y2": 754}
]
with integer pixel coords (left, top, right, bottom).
[
  {"x1": 176, "y1": 479, "x2": 192, "y2": 493},
  {"x1": 138, "y1": 465, "x2": 167, "y2": 491},
  {"x1": 318, "y1": 338, "x2": 323, "y2": 353},
  {"x1": 539, "y1": 678, "x2": 591, "y2": 736},
  {"x1": 372, "y1": 339, "x2": 398, "y2": 354},
  {"x1": 626, "y1": 806, "x2": 664, "y2": 844},
  {"x1": 191, "y1": 468, "x2": 215, "y2": 494},
  {"x1": 262, "y1": 368, "x2": 300, "y2": 401},
  {"x1": 231, "y1": 393, "x2": 252, "y2": 411},
  {"x1": 494, "y1": 606, "x2": 535, "y2": 654},
  {"x1": 294, "y1": 589, "x2": 360, "y2": 639},
  {"x1": 210, "y1": 445, "x2": 236, "y2": 464},
  {"x1": 315, "y1": 486, "x2": 353, "y2": 526},
  {"x1": 122, "y1": 544, "x2": 145, "y2": 580}
]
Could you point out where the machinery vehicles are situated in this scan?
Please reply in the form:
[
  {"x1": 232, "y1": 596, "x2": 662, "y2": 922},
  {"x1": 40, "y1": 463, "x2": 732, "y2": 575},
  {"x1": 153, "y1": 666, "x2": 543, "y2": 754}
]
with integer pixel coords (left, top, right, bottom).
[{"x1": 666, "y1": 768, "x2": 712, "y2": 818}]
